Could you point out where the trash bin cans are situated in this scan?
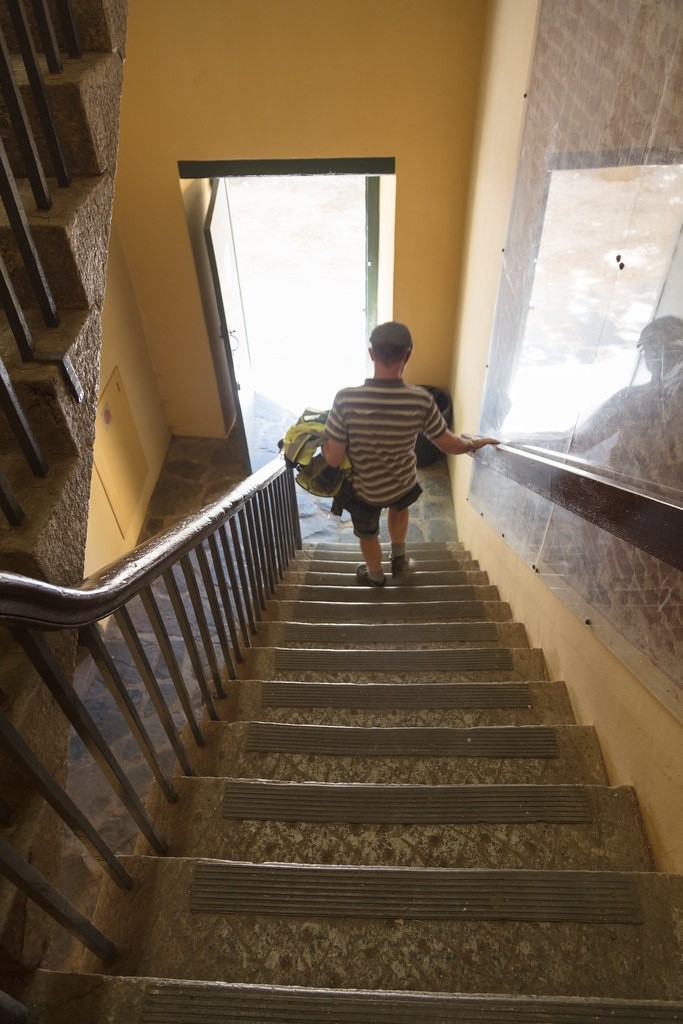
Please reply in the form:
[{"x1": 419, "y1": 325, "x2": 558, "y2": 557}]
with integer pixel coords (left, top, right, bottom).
[{"x1": 414, "y1": 385, "x2": 451, "y2": 468}]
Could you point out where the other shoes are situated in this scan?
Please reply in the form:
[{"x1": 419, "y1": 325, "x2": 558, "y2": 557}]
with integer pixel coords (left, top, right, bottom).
[
  {"x1": 358, "y1": 561, "x2": 386, "y2": 586},
  {"x1": 389, "y1": 550, "x2": 409, "y2": 564}
]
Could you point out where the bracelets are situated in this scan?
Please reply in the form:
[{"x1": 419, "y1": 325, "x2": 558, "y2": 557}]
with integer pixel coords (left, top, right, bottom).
[{"x1": 468, "y1": 440, "x2": 476, "y2": 452}]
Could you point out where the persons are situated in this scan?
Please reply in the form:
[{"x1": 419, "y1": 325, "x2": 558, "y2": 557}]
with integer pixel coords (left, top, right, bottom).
[
  {"x1": 516, "y1": 315, "x2": 683, "y2": 489},
  {"x1": 320, "y1": 322, "x2": 500, "y2": 588}
]
